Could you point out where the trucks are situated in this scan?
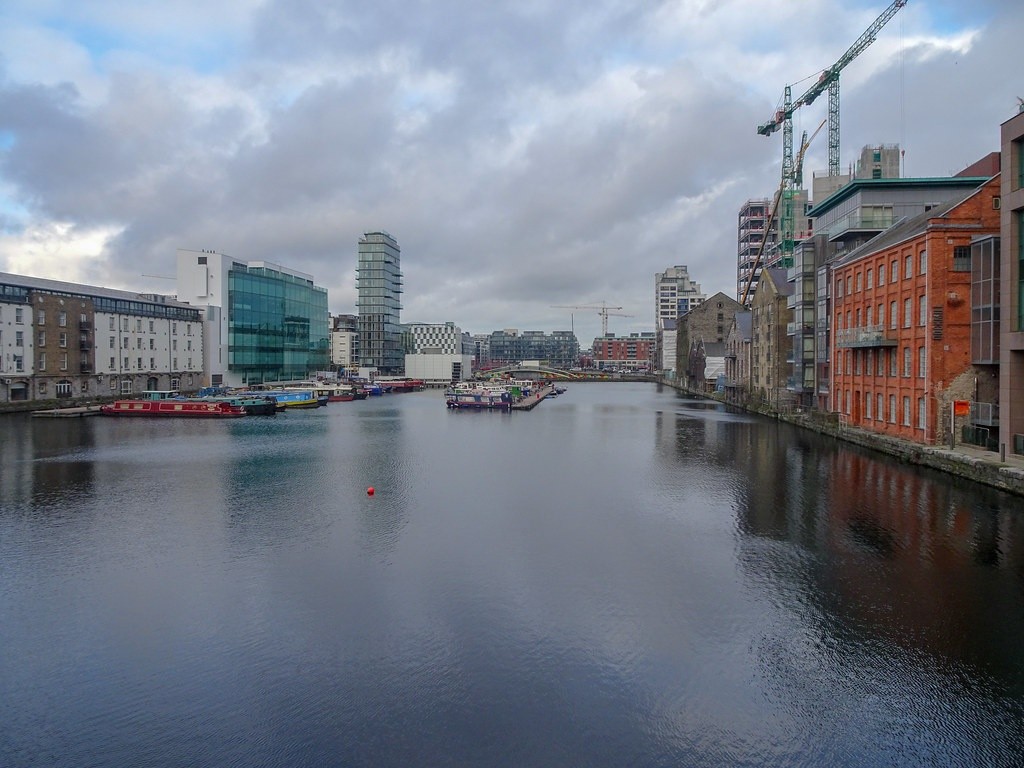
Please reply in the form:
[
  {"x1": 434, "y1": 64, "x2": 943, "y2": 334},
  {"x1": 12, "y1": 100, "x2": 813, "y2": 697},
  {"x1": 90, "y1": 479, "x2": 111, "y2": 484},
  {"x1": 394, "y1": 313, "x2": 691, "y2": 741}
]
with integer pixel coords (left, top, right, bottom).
[{"x1": 519, "y1": 360, "x2": 539, "y2": 367}]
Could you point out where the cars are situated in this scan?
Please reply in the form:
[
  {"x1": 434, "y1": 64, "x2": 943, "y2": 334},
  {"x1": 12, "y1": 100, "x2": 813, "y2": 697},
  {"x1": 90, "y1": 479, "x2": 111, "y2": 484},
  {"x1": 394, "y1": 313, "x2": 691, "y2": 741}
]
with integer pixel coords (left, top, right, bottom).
[
  {"x1": 618, "y1": 369, "x2": 631, "y2": 374},
  {"x1": 569, "y1": 367, "x2": 582, "y2": 372}
]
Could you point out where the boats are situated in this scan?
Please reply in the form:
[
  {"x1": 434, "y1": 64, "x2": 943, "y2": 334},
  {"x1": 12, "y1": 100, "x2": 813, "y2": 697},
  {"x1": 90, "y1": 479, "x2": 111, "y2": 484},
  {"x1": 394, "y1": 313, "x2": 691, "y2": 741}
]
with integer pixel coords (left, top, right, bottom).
[
  {"x1": 100, "y1": 378, "x2": 392, "y2": 416},
  {"x1": 444, "y1": 379, "x2": 538, "y2": 408}
]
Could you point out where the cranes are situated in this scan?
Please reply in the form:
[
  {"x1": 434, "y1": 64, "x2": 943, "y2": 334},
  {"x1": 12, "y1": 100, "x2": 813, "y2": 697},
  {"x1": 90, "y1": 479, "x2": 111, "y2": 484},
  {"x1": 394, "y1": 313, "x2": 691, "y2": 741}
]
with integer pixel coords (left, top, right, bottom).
[
  {"x1": 756, "y1": 0, "x2": 908, "y2": 268},
  {"x1": 549, "y1": 298, "x2": 633, "y2": 337}
]
